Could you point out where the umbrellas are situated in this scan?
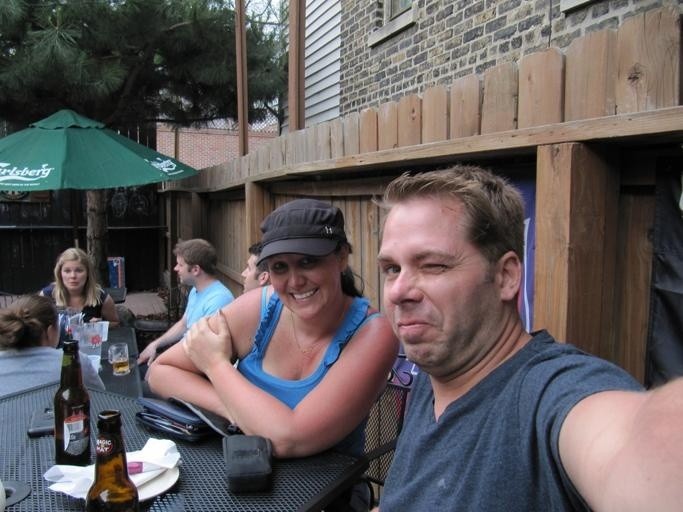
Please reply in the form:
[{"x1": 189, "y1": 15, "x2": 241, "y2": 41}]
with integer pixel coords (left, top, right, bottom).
[{"x1": 0, "y1": 107, "x2": 200, "y2": 248}]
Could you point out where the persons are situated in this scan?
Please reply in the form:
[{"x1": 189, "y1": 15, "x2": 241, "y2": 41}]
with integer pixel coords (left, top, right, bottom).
[
  {"x1": 0, "y1": 293, "x2": 107, "y2": 397},
  {"x1": 36, "y1": 248, "x2": 120, "y2": 329},
  {"x1": 145, "y1": 197, "x2": 402, "y2": 510},
  {"x1": 238, "y1": 240, "x2": 274, "y2": 297},
  {"x1": 369, "y1": 162, "x2": 683, "y2": 512},
  {"x1": 126, "y1": 239, "x2": 235, "y2": 405}
]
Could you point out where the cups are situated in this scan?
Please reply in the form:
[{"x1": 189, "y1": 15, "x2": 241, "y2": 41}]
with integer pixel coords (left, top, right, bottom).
[
  {"x1": 97, "y1": 322, "x2": 110, "y2": 341},
  {"x1": 107, "y1": 342, "x2": 130, "y2": 377}
]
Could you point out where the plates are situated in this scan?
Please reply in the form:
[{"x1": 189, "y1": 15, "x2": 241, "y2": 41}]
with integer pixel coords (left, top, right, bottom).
[{"x1": 127, "y1": 451, "x2": 180, "y2": 503}]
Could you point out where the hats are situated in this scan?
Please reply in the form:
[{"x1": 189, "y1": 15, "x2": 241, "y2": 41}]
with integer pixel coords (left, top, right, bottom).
[{"x1": 254, "y1": 197, "x2": 345, "y2": 267}]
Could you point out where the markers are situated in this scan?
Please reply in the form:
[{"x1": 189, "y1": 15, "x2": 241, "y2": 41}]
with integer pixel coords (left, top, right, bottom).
[{"x1": 126, "y1": 459, "x2": 182, "y2": 474}]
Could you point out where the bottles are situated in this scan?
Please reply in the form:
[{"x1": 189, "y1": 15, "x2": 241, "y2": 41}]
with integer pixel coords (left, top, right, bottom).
[
  {"x1": 83, "y1": 408, "x2": 141, "y2": 511},
  {"x1": 52, "y1": 339, "x2": 92, "y2": 466}
]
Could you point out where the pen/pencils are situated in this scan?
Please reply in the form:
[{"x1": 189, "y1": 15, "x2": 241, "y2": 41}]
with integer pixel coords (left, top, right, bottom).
[{"x1": 139, "y1": 406, "x2": 194, "y2": 436}]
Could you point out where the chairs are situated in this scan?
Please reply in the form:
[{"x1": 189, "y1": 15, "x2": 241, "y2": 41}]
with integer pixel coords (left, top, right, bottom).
[
  {"x1": 134, "y1": 288, "x2": 179, "y2": 343},
  {"x1": 365, "y1": 367, "x2": 415, "y2": 510}
]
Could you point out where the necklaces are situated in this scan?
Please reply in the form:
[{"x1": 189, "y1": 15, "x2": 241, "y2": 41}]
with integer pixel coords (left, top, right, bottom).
[{"x1": 289, "y1": 297, "x2": 347, "y2": 354}]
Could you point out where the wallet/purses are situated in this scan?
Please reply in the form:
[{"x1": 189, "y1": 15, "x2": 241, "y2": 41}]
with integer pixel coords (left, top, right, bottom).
[{"x1": 134, "y1": 396, "x2": 203, "y2": 445}]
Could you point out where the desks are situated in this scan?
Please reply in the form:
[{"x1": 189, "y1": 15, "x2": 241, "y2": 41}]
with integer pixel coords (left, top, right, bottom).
[
  {"x1": 0, "y1": 378, "x2": 370, "y2": 512},
  {"x1": 100, "y1": 326, "x2": 143, "y2": 397},
  {"x1": 102, "y1": 287, "x2": 128, "y2": 304}
]
